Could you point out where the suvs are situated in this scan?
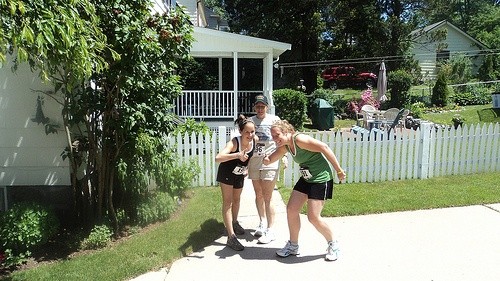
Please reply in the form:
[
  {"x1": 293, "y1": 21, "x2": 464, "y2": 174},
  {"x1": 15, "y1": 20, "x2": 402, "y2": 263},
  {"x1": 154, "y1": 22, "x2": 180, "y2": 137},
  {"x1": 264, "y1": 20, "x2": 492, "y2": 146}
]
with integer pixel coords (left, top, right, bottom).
[{"x1": 321, "y1": 66, "x2": 378, "y2": 90}]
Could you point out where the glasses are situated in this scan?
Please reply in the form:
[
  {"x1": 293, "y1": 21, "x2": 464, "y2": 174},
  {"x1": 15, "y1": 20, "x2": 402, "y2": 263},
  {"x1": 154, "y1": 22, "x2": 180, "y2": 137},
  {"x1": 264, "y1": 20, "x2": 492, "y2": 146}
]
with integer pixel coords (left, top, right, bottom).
[{"x1": 255, "y1": 106, "x2": 266, "y2": 110}]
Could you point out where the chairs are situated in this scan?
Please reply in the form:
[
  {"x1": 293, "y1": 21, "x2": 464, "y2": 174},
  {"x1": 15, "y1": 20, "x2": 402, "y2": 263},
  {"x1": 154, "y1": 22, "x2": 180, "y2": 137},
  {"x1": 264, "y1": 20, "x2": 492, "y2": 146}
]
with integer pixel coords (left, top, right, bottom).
[{"x1": 357, "y1": 105, "x2": 411, "y2": 130}]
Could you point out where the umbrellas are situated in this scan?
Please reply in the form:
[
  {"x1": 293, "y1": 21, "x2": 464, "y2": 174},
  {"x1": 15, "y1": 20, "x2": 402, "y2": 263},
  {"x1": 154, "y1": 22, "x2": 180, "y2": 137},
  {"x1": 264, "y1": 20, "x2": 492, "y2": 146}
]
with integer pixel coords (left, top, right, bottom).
[{"x1": 377, "y1": 60, "x2": 388, "y2": 100}]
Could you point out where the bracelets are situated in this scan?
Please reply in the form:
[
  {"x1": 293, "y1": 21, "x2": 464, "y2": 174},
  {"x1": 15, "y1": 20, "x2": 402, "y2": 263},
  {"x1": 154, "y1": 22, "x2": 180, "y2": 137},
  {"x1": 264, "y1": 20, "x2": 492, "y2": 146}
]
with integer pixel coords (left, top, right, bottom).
[{"x1": 338, "y1": 170, "x2": 343, "y2": 174}]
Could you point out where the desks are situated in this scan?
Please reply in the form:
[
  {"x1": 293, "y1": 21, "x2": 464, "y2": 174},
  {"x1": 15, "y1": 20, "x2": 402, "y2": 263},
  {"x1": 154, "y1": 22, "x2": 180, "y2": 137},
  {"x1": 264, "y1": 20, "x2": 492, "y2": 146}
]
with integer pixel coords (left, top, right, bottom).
[{"x1": 368, "y1": 109, "x2": 385, "y2": 129}]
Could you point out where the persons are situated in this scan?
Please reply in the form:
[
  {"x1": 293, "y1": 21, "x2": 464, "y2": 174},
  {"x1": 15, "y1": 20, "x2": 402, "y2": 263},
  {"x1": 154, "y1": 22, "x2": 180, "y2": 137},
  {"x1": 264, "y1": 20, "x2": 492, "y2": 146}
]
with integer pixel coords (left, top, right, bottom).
[
  {"x1": 248, "y1": 94, "x2": 281, "y2": 244},
  {"x1": 216, "y1": 114, "x2": 259, "y2": 251},
  {"x1": 262, "y1": 120, "x2": 346, "y2": 260}
]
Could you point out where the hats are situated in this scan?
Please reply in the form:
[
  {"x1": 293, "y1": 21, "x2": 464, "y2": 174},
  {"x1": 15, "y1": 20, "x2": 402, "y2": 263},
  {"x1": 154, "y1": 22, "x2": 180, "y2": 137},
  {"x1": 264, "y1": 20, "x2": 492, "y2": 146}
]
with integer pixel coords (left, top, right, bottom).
[{"x1": 254, "y1": 95, "x2": 268, "y2": 106}]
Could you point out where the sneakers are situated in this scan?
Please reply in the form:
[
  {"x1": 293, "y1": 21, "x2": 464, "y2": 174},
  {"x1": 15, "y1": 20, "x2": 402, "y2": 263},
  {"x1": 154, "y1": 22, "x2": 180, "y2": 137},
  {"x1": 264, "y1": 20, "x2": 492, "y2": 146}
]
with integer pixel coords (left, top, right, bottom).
[
  {"x1": 233, "y1": 221, "x2": 244, "y2": 235},
  {"x1": 254, "y1": 223, "x2": 267, "y2": 236},
  {"x1": 227, "y1": 234, "x2": 245, "y2": 251},
  {"x1": 325, "y1": 241, "x2": 339, "y2": 261},
  {"x1": 276, "y1": 241, "x2": 299, "y2": 257},
  {"x1": 258, "y1": 229, "x2": 275, "y2": 243}
]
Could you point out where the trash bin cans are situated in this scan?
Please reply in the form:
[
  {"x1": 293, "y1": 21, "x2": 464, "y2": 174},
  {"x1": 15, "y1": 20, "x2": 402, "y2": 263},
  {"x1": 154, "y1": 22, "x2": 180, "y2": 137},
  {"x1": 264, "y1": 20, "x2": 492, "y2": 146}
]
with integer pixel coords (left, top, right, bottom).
[{"x1": 310, "y1": 98, "x2": 335, "y2": 131}]
